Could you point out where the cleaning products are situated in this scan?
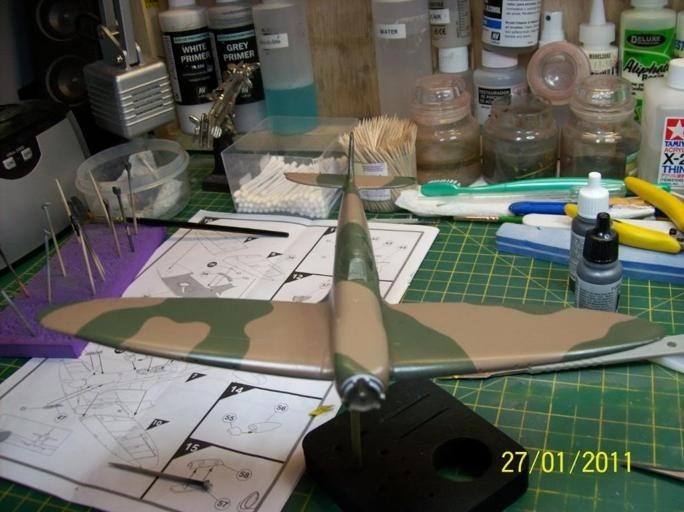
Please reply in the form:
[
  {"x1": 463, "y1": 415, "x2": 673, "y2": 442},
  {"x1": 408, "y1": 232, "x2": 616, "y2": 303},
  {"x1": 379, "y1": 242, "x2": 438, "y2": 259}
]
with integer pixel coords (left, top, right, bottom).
[
  {"x1": 618, "y1": 0, "x2": 684, "y2": 193},
  {"x1": 158, "y1": 0, "x2": 319, "y2": 135}
]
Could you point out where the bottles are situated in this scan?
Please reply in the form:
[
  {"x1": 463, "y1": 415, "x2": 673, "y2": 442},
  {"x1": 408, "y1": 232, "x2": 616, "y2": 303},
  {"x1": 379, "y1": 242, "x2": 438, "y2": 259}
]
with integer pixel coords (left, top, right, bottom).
[
  {"x1": 157, "y1": 0, "x2": 223, "y2": 137},
  {"x1": 566, "y1": 171, "x2": 611, "y2": 292},
  {"x1": 253, "y1": 0, "x2": 320, "y2": 137},
  {"x1": 575, "y1": 213, "x2": 625, "y2": 315},
  {"x1": 371, "y1": 0, "x2": 684, "y2": 192},
  {"x1": 206, "y1": 0, "x2": 269, "y2": 134}
]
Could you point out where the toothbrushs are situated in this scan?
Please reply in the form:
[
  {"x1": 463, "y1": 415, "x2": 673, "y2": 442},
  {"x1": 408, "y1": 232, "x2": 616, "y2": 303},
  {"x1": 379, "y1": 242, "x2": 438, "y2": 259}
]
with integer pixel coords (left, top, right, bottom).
[{"x1": 420, "y1": 178, "x2": 672, "y2": 199}]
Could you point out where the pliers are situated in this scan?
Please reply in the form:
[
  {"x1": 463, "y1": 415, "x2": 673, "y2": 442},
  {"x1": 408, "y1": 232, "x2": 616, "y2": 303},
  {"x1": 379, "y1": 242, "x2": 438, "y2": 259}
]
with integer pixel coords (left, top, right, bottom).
[{"x1": 563, "y1": 176, "x2": 684, "y2": 253}]
